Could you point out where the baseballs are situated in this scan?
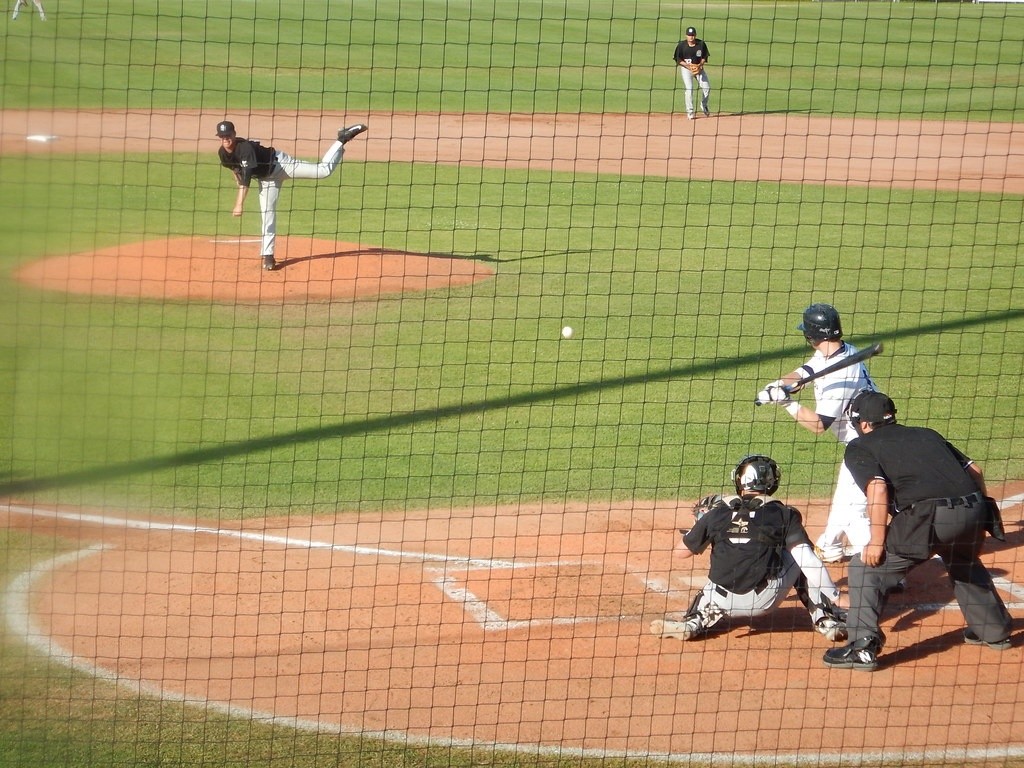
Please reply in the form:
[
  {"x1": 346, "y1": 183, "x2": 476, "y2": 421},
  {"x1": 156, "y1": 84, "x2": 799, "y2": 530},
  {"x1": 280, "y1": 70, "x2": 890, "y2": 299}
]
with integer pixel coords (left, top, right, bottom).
[{"x1": 562, "y1": 327, "x2": 574, "y2": 339}]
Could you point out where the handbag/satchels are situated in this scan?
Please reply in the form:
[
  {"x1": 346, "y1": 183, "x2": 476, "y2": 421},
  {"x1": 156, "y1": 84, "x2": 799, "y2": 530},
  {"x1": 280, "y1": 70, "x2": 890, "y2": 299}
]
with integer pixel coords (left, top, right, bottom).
[
  {"x1": 883, "y1": 500, "x2": 935, "y2": 560},
  {"x1": 981, "y1": 495, "x2": 1006, "y2": 542}
]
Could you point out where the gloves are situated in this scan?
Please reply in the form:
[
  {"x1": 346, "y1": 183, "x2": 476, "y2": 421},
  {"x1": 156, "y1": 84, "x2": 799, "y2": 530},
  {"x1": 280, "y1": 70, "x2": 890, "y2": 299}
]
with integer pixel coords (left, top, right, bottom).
[{"x1": 757, "y1": 380, "x2": 800, "y2": 416}]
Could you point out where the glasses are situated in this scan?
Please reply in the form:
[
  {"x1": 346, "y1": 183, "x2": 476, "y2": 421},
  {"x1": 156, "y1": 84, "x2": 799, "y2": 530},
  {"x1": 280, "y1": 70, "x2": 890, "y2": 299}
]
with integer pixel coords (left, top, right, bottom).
[{"x1": 688, "y1": 34, "x2": 694, "y2": 36}]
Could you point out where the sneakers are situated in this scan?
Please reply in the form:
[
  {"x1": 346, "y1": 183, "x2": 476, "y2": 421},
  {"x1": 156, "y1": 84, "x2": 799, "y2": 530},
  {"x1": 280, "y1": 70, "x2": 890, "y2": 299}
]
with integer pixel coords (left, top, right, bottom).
[
  {"x1": 962, "y1": 627, "x2": 1012, "y2": 650},
  {"x1": 338, "y1": 124, "x2": 368, "y2": 142},
  {"x1": 822, "y1": 640, "x2": 878, "y2": 672},
  {"x1": 262, "y1": 255, "x2": 274, "y2": 270}
]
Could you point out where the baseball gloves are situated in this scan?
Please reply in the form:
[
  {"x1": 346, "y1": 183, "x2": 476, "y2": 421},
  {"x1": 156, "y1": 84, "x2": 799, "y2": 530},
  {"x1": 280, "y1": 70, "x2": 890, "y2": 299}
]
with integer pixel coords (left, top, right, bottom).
[
  {"x1": 689, "y1": 63, "x2": 701, "y2": 74},
  {"x1": 692, "y1": 495, "x2": 723, "y2": 523}
]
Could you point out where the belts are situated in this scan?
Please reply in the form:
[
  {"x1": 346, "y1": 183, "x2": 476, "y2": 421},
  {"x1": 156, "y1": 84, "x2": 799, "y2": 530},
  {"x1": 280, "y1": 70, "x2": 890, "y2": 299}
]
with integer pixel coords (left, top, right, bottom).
[{"x1": 935, "y1": 494, "x2": 977, "y2": 508}]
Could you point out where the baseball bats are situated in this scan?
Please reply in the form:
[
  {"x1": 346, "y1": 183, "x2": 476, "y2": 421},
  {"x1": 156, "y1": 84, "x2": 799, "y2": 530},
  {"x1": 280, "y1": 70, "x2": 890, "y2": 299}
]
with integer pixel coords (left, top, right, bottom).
[{"x1": 753, "y1": 342, "x2": 885, "y2": 407}]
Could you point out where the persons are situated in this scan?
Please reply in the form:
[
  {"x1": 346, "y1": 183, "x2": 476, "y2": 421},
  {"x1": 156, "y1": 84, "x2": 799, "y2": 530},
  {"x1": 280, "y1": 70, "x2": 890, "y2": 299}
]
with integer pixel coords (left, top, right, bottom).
[
  {"x1": 216, "y1": 121, "x2": 368, "y2": 270},
  {"x1": 650, "y1": 453, "x2": 849, "y2": 640},
  {"x1": 672, "y1": 27, "x2": 711, "y2": 120},
  {"x1": 757, "y1": 302, "x2": 907, "y2": 590},
  {"x1": 822, "y1": 392, "x2": 1015, "y2": 671}
]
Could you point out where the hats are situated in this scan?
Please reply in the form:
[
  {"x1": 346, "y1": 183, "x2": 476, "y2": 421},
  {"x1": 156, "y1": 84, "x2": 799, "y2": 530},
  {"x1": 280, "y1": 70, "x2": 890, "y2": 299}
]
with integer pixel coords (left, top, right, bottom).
[{"x1": 849, "y1": 391, "x2": 897, "y2": 426}]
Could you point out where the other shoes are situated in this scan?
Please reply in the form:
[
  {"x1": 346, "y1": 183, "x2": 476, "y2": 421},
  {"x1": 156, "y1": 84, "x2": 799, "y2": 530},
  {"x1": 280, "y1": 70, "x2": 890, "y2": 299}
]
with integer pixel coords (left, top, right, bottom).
[
  {"x1": 812, "y1": 541, "x2": 844, "y2": 564},
  {"x1": 688, "y1": 113, "x2": 693, "y2": 119},
  {"x1": 649, "y1": 618, "x2": 701, "y2": 641},
  {"x1": 813, "y1": 617, "x2": 846, "y2": 638},
  {"x1": 701, "y1": 103, "x2": 709, "y2": 115},
  {"x1": 892, "y1": 578, "x2": 906, "y2": 590}
]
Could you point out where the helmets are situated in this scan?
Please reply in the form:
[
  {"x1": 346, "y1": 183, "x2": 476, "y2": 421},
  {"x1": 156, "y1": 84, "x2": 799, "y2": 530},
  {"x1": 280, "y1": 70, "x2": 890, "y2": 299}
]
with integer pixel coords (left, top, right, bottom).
[
  {"x1": 736, "y1": 456, "x2": 781, "y2": 500},
  {"x1": 796, "y1": 304, "x2": 843, "y2": 342},
  {"x1": 687, "y1": 27, "x2": 695, "y2": 35},
  {"x1": 215, "y1": 121, "x2": 234, "y2": 135}
]
